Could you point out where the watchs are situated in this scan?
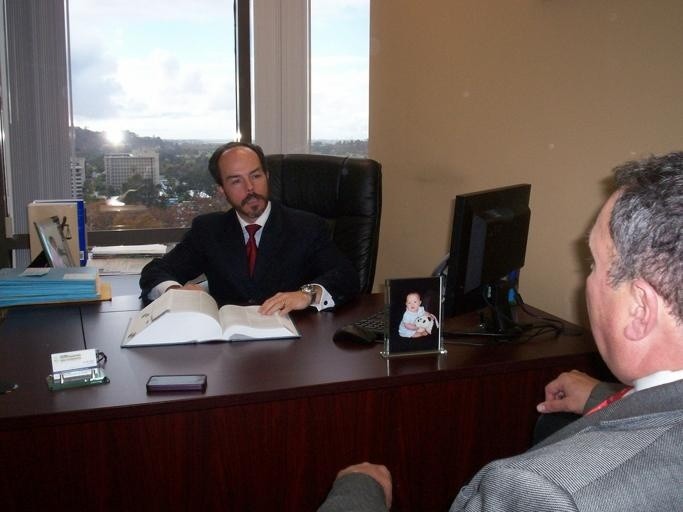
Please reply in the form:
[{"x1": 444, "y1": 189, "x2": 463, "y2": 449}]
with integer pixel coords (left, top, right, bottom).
[{"x1": 299, "y1": 282, "x2": 316, "y2": 304}]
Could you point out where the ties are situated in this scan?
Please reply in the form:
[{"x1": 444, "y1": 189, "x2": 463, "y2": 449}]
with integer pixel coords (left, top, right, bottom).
[
  {"x1": 573, "y1": 383, "x2": 638, "y2": 417},
  {"x1": 244, "y1": 224, "x2": 263, "y2": 282}
]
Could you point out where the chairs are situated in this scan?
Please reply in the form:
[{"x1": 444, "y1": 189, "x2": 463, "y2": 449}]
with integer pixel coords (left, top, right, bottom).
[{"x1": 262, "y1": 153, "x2": 381, "y2": 294}]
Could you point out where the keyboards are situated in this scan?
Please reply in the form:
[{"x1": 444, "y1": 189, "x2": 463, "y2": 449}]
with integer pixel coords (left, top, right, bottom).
[{"x1": 355, "y1": 309, "x2": 386, "y2": 340}]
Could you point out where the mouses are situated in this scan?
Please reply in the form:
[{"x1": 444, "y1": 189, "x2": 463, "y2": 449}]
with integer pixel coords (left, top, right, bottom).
[{"x1": 333, "y1": 324, "x2": 375, "y2": 344}]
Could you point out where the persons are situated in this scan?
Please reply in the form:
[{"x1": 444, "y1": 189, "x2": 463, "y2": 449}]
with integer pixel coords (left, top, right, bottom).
[
  {"x1": 311, "y1": 146, "x2": 683, "y2": 512},
  {"x1": 139, "y1": 141, "x2": 363, "y2": 319},
  {"x1": 397, "y1": 291, "x2": 435, "y2": 339}
]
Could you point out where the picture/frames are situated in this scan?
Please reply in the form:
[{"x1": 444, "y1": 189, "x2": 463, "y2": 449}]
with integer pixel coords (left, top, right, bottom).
[{"x1": 28, "y1": 215, "x2": 76, "y2": 268}]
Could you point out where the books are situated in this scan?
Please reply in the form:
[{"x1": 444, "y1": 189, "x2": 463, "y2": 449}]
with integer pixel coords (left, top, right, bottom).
[
  {"x1": 118, "y1": 288, "x2": 303, "y2": 348},
  {"x1": 0, "y1": 199, "x2": 101, "y2": 308}
]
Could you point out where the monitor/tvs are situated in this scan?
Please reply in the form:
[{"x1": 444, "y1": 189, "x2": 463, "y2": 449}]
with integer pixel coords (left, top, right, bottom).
[{"x1": 443, "y1": 183, "x2": 531, "y2": 337}]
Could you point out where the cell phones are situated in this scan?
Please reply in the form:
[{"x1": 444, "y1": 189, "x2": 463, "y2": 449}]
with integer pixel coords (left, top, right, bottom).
[{"x1": 146, "y1": 374, "x2": 207, "y2": 391}]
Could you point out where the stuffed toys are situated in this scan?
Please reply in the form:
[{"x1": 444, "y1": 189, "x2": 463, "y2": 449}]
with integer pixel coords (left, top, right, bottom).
[{"x1": 415, "y1": 314, "x2": 437, "y2": 339}]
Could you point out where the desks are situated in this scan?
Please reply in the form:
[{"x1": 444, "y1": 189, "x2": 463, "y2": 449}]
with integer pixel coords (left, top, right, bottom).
[{"x1": 0, "y1": 294, "x2": 607, "y2": 512}]
